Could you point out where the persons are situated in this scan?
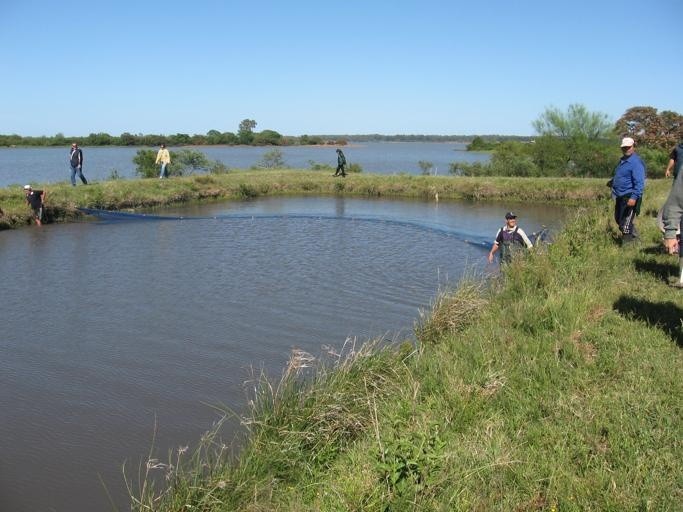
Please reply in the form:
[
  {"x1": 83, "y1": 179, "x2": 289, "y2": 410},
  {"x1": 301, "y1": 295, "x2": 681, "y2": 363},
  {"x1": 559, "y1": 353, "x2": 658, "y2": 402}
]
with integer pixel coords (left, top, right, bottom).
[
  {"x1": 70, "y1": 143, "x2": 87, "y2": 186},
  {"x1": 612, "y1": 137, "x2": 645, "y2": 244},
  {"x1": 488, "y1": 212, "x2": 534, "y2": 266},
  {"x1": 332, "y1": 149, "x2": 346, "y2": 178},
  {"x1": 665, "y1": 142, "x2": 683, "y2": 186},
  {"x1": 662, "y1": 165, "x2": 683, "y2": 289},
  {"x1": 155, "y1": 143, "x2": 171, "y2": 179},
  {"x1": 24, "y1": 185, "x2": 46, "y2": 226}
]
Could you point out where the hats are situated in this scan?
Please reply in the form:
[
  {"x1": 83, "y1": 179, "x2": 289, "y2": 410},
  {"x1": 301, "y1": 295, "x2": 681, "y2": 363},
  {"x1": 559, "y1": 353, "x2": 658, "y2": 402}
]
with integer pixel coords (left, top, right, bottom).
[
  {"x1": 505, "y1": 212, "x2": 517, "y2": 220},
  {"x1": 620, "y1": 136, "x2": 634, "y2": 148}
]
